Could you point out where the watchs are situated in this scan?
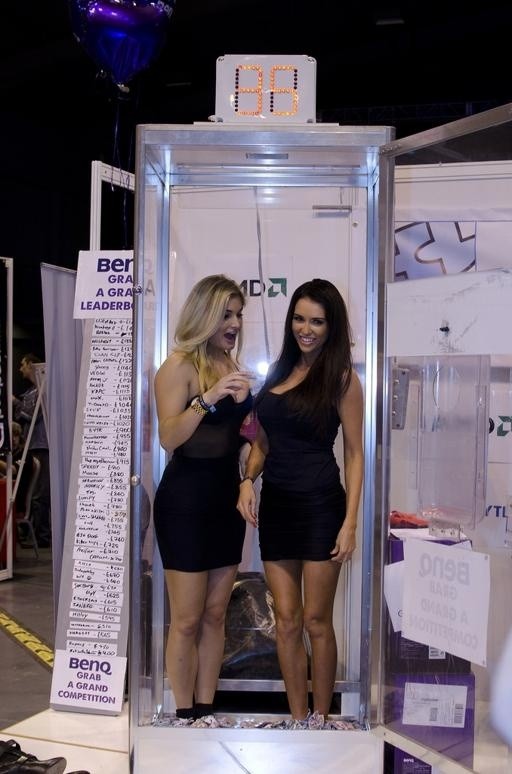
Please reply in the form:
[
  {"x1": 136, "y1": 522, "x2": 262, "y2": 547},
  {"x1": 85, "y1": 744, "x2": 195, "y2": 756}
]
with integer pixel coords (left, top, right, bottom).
[{"x1": 191, "y1": 396, "x2": 208, "y2": 415}]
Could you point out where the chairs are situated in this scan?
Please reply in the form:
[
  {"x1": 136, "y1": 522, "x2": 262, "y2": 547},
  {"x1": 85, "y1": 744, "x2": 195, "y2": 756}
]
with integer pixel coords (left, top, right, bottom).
[{"x1": 16, "y1": 456, "x2": 40, "y2": 559}]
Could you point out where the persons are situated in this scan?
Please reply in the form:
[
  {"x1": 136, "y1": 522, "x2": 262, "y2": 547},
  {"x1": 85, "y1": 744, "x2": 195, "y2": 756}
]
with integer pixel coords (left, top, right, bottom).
[
  {"x1": 1, "y1": 420, "x2": 33, "y2": 512},
  {"x1": 236, "y1": 279, "x2": 365, "y2": 727},
  {"x1": 150, "y1": 273, "x2": 259, "y2": 718},
  {"x1": 13, "y1": 353, "x2": 52, "y2": 548}
]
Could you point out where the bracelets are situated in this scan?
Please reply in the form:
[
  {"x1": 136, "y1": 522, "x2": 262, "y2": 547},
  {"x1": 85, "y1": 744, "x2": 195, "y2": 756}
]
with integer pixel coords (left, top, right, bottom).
[
  {"x1": 241, "y1": 477, "x2": 255, "y2": 482},
  {"x1": 199, "y1": 394, "x2": 216, "y2": 413}
]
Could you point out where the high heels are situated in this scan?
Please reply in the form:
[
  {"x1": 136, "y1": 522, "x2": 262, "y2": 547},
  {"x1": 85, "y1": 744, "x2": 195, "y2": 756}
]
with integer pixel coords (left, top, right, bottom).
[{"x1": 0, "y1": 739, "x2": 90, "y2": 774}]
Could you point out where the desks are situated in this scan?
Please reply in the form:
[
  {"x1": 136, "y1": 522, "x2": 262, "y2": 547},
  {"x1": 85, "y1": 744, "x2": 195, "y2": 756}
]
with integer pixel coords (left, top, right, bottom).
[{"x1": 0, "y1": 479, "x2": 16, "y2": 582}]
